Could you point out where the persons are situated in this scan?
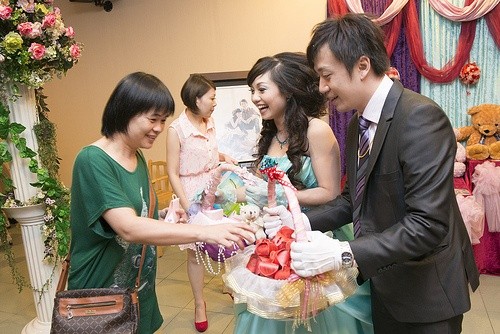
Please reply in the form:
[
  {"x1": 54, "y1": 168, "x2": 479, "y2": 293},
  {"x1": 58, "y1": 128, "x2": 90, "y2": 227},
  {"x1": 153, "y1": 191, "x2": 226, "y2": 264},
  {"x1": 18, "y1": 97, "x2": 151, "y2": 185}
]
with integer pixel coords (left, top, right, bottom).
[
  {"x1": 166, "y1": 75, "x2": 238, "y2": 332},
  {"x1": 68, "y1": 71, "x2": 257, "y2": 334},
  {"x1": 263, "y1": 14, "x2": 480, "y2": 334},
  {"x1": 201, "y1": 52, "x2": 374, "y2": 334}
]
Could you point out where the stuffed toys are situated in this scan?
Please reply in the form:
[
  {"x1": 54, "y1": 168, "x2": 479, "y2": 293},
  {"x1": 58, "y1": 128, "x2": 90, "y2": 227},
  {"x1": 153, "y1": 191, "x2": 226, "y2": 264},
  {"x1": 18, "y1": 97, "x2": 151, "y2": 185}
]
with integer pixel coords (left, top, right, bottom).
[
  {"x1": 457, "y1": 103, "x2": 500, "y2": 160},
  {"x1": 453, "y1": 129, "x2": 466, "y2": 177},
  {"x1": 240, "y1": 205, "x2": 266, "y2": 245}
]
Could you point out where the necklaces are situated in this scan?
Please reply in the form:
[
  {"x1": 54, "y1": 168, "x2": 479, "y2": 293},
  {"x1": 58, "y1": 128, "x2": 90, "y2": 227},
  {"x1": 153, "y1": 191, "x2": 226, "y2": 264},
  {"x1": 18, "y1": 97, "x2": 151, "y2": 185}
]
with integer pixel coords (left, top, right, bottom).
[{"x1": 276, "y1": 135, "x2": 289, "y2": 149}]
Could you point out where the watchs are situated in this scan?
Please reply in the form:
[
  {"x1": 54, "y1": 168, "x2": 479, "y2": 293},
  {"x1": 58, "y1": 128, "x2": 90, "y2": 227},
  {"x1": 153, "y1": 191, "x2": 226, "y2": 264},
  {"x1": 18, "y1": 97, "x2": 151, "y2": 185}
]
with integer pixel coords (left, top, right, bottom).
[{"x1": 341, "y1": 242, "x2": 353, "y2": 267}]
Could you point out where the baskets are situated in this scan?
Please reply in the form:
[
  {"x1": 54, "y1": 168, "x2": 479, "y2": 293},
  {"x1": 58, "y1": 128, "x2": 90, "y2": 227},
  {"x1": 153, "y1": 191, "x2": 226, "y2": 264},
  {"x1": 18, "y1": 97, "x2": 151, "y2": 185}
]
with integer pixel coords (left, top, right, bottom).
[
  {"x1": 189, "y1": 164, "x2": 267, "y2": 263},
  {"x1": 221, "y1": 166, "x2": 360, "y2": 322}
]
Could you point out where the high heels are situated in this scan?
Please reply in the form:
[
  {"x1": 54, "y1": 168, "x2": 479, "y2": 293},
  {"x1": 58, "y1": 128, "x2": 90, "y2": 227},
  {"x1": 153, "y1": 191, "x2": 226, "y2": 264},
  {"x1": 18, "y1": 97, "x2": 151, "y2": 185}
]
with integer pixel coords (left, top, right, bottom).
[{"x1": 195, "y1": 301, "x2": 208, "y2": 332}]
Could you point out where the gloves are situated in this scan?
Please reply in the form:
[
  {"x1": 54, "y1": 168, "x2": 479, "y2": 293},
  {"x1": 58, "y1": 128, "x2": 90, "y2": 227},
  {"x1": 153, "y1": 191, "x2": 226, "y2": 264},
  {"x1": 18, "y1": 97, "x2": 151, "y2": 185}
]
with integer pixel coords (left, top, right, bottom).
[
  {"x1": 262, "y1": 205, "x2": 311, "y2": 239},
  {"x1": 290, "y1": 230, "x2": 354, "y2": 277}
]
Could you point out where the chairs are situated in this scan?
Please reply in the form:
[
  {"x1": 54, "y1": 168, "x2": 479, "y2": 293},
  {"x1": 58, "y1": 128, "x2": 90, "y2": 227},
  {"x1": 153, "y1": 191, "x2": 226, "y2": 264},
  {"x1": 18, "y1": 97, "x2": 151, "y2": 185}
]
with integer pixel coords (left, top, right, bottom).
[
  {"x1": 153, "y1": 175, "x2": 173, "y2": 211},
  {"x1": 147, "y1": 158, "x2": 167, "y2": 192}
]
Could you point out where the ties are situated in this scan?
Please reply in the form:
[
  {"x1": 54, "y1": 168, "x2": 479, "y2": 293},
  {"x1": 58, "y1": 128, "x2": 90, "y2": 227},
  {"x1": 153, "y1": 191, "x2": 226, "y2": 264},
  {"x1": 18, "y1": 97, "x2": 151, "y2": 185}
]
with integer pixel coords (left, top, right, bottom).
[{"x1": 353, "y1": 115, "x2": 371, "y2": 239}]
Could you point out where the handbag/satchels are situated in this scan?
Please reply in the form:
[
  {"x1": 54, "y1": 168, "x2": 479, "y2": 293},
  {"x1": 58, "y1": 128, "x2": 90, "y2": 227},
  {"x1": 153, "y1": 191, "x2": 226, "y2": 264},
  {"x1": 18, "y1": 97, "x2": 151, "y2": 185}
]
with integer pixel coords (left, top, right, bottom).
[{"x1": 50, "y1": 285, "x2": 140, "y2": 334}]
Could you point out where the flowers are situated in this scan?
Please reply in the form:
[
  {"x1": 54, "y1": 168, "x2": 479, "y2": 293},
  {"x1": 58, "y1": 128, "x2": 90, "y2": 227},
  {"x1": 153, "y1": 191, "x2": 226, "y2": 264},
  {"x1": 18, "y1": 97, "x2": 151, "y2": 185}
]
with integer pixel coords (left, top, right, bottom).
[{"x1": 0, "y1": 0, "x2": 83, "y2": 87}]
